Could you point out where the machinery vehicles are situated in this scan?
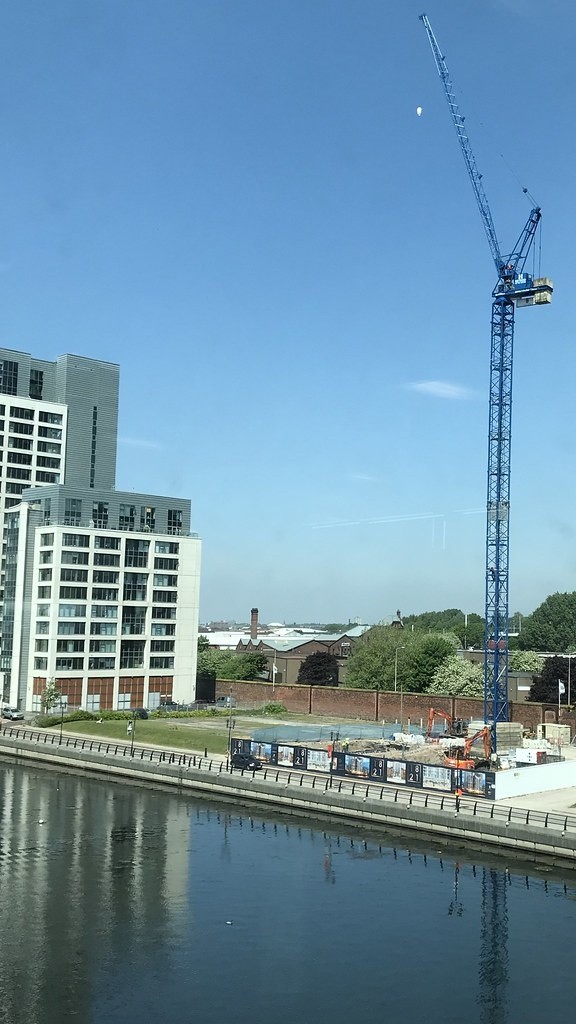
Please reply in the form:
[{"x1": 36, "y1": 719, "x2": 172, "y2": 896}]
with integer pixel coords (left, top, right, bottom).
[
  {"x1": 424, "y1": 707, "x2": 472, "y2": 743},
  {"x1": 443, "y1": 727, "x2": 493, "y2": 771}
]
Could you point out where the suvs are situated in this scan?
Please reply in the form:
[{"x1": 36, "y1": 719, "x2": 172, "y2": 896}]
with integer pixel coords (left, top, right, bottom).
[
  {"x1": 3, "y1": 707, "x2": 24, "y2": 721},
  {"x1": 157, "y1": 701, "x2": 186, "y2": 712},
  {"x1": 133, "y1": 707, "x2": 148, "y2": 720},
  {"x1": 215, "y1": 696, "x2": 236, "y2": 708},
  {"x1": 230, "y1": 753, "x2": 264, "y2": 771},
  {"x1": 185, "y1": 700, "x2": 208, "y2": 710}
]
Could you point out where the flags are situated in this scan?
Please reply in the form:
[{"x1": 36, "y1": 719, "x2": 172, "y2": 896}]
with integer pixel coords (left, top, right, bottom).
[
  {"x1": 559, "y1": 683, "x2": 565, "y2": 693},
  {"x1": 273, "y1": 666, "x2": 277, "y2": 673}
]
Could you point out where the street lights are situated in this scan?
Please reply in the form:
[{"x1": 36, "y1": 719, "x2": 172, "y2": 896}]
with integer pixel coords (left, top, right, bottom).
[
  {"x1": 130, "y1": 709, "x2": 140, "y2": 757},
  {"x1": 395, "y1": 646, "x2": 406, "y2": 691},
  {"x1": 60, "y1": 701, "x2": 67, "y2": 746},
  {"x1": 226, "y1": 719, "x2": 236, "y2": 771},
  {"x1": 329, "y1": 732, "x2": 339, "y2": 789},
  {"x1": 452, "y1": 747, "x2": 461, "y2": 810}
]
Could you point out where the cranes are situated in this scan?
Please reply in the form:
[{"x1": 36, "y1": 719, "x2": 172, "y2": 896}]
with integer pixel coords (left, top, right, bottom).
[{"x1": 418, "y1": 13, "x2": 556, "y2": 723}]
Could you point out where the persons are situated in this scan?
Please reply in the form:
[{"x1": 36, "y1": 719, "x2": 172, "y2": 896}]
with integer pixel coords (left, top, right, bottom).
[
  {"x1": 127, "y1": 721, "x2": 132, "y2": 735},
  {"x1": 455, "y1": 786, "x2": 462, "y2": 809}
]
